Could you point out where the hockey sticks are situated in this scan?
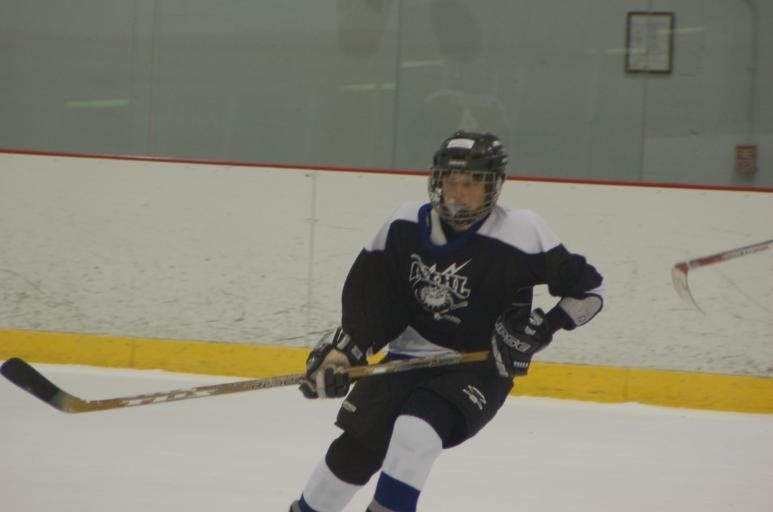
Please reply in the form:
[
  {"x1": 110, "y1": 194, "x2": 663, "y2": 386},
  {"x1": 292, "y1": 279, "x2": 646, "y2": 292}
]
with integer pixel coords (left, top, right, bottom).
[
  {"x1": 0, "y1": 351, "x2": 489, "y2": 413},
  {"x1": 671, "y1": 239, "x2": 773, "y2": 312}
]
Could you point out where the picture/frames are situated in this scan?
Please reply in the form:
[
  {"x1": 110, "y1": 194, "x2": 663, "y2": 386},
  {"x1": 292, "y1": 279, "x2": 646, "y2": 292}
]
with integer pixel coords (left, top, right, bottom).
[{"x1": 624, "y1": 12, "x2": 674, "y2": 75}]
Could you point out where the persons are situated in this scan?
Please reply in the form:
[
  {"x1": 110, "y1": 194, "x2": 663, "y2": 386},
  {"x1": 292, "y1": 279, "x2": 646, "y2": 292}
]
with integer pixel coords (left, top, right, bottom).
[{"x1": 289, "y1": 129, "x2": 604, "y2": 512}]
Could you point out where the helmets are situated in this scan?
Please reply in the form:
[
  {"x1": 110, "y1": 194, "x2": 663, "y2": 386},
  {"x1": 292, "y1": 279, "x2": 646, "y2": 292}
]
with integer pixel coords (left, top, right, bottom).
[{"x1": 428, "y1": 129, "x2": 508, "y2": 230}]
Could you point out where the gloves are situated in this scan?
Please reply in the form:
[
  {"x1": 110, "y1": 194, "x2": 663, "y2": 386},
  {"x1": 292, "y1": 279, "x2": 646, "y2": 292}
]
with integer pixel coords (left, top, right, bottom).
[
  {"x1": 491, "y1": 307, "x2": 552, "y2": 383},
  {"x1": 298, "y1": 327, "x2": 364, "y2": 398}
]
